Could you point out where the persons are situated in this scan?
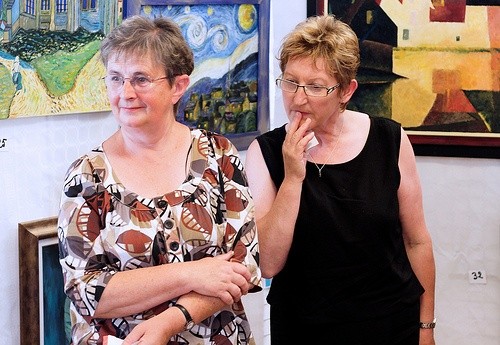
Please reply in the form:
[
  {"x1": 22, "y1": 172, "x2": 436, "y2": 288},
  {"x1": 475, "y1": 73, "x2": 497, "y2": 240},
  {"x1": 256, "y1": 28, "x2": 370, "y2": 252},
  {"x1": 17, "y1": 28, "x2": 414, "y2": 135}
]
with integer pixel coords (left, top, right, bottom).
[
  {"x1": 245, "y1": 14, "x2": 435, "y2": 345},
  {"x1": 57, "y1": 13, "x2": 263, "y2": 345}
]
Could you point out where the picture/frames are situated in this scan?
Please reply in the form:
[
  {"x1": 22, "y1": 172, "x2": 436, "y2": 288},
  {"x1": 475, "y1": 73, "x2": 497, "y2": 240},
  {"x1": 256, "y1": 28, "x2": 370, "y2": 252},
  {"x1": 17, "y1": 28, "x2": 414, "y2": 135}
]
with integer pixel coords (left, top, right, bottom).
[
  {"x1": 307, "y1": 0, "x2": 500, "y2": 160},
  {"x1": 123, "y1": 0, "x2": 270, "y2": 151},
  {"x1": 18, "y1": 216, "x2": 68, "y2": 345}
]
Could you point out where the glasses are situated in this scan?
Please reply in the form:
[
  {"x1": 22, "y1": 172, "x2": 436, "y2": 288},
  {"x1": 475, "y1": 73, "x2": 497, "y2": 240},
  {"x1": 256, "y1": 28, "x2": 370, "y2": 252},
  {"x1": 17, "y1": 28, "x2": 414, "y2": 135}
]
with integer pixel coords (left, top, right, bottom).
[
  {"x1": 275, "y1": 73, "x2": 341, "y2": 97},
  {"x1": 102, "y1": 73, "x2": 181, "y2": 91}
]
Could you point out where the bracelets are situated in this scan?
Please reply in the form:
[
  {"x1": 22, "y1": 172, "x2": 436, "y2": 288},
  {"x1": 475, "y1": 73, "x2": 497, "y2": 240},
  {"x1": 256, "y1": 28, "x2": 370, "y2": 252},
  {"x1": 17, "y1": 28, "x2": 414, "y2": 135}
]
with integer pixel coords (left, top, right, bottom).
[{"x1": 420, "y1": 317, "x2": 437, "y2": 329}]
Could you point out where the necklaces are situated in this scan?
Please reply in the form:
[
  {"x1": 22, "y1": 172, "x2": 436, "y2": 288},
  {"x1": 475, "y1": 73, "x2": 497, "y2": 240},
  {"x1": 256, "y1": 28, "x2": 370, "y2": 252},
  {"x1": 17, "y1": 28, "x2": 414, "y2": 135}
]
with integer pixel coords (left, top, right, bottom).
[{"x1": 307, "y1": 120, "x2": 344, "y2": 177}]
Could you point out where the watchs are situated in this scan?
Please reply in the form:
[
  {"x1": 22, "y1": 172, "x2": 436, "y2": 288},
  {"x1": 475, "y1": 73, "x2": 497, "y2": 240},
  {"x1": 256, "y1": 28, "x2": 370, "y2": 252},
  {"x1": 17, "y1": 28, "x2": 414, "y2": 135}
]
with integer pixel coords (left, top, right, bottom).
[{"x1": 172, "y1": 304, "x2": 195, "y2": 331}]
